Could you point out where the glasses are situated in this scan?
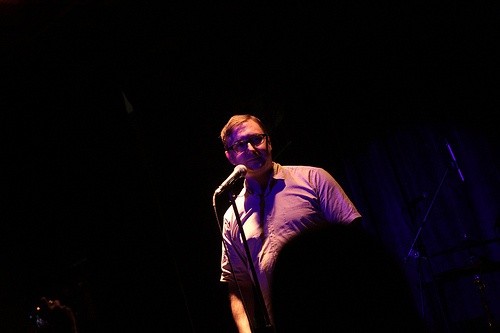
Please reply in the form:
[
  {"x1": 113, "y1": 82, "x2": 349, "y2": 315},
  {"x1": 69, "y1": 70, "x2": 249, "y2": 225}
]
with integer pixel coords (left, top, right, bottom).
[{"x1": 226, "y1": 134, "x2": 270, "y2": 151}]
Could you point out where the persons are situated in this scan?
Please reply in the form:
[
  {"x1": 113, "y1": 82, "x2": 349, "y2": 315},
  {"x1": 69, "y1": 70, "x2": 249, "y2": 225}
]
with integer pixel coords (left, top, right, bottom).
[
  {"x1": 220, "y1": 115, "x2": 364, "y2": 333},
  {"x1": 260, "y1": 218, "x2": 419, "y2": 333}
]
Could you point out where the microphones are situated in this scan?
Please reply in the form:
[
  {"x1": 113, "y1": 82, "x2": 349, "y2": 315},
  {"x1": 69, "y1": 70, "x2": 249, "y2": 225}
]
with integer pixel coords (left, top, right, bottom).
[
  {"x1": 215, "y1": 164, "x2": 248, "y2": 195},
  {"x1": 447, "y1": 144, "x2": 465, "y2": 181}
]
